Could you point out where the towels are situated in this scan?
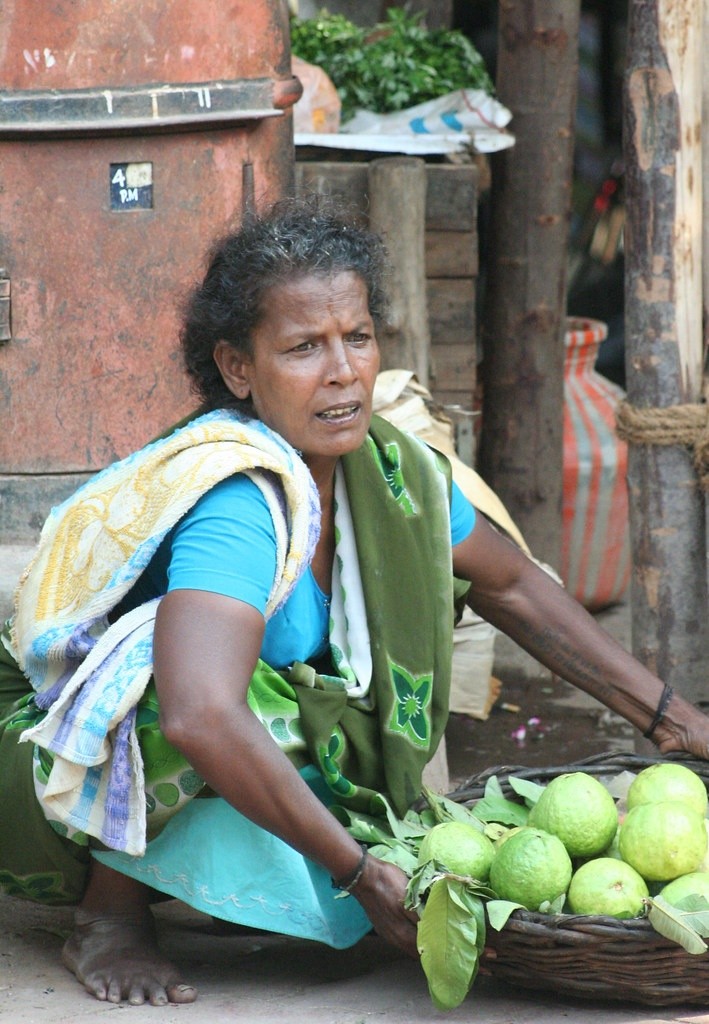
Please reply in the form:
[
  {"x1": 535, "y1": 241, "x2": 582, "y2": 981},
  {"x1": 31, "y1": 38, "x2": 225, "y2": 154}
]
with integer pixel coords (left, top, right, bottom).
[{"x1": 0, "y1": 405, "x2": 325, "y2": 861}]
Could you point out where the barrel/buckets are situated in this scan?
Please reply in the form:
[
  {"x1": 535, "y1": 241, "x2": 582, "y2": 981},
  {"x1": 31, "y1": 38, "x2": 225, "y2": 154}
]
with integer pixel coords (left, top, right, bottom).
[{"x1": 567, "y1": 317, "x2": 638, "y2": 618}]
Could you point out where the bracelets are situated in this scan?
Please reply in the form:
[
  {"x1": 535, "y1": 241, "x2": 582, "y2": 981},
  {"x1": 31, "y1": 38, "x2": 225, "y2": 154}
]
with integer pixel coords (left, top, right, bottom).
[
  {"x1": 330, "y1": 842, "x2": 369, "y2": 890},
  {"x1": 643, "y1": 683, "x2": 673, "y2": 740}
]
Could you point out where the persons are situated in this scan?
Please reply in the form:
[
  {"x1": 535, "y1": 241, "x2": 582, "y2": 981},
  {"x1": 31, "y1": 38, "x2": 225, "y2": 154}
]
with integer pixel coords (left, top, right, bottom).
[{"x1": 30, "y1": 193, "x2": 709, "y2": 1009}]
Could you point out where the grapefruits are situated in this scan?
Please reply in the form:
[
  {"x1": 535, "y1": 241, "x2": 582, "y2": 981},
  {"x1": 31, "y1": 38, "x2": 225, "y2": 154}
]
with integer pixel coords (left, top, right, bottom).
[{"x1": 416, "y1": 761, "x2": 707, "y2": 917}]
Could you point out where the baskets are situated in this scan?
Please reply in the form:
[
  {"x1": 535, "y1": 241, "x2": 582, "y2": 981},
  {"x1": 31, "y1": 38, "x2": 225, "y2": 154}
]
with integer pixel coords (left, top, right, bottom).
[{"x1": 428, "y1": 756, "x2": 707, "y2": 1007}]
